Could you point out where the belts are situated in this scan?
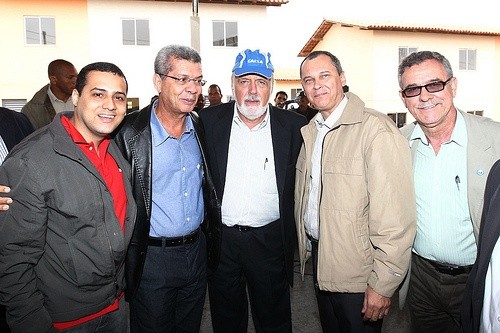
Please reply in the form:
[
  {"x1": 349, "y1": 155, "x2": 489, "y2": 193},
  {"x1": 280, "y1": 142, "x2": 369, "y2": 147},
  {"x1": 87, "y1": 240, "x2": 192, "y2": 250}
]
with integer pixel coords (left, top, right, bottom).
[
  {"x1": 235, "y1": 225, "x2": 253, "y2": 232},
  {"x1": 430, "y1": 261, "x2": 473, "y2": 275},
  {"x1": 147, "y1": 221, "x2": 208, "y2": 247}
]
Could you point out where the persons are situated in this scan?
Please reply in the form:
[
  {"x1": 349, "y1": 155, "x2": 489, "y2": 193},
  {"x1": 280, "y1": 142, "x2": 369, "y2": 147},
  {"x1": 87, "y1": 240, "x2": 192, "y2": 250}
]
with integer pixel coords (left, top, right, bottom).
[
  {"x1": 197, "y1": 49, "x2": 309, "y2": 333},
  {"x1": 0, "y1": 108, "x2": 34, "y2": 212},
  {"x1": 395, "y1": 51, "x2": 500, "y2": 333},
  {"x1": 294, "y1": 90, "x2": 319, "y2": 119},
  {"x1": 294, "y1": 51, "x2": 417, "y2": 333},
  {"x1": 192, "y1": 94, "x2": 209, "y2": 111},
  {"x1": 466, "y1": 160, "x2": 500, "y2": 333},
  {"x1": 207, "y1": 83, "x2": 223, "y2": 106},
  {"x1": 275, "y1": 90, "x2": 289, "y2": 109},
  {"x1": 0, "y1": 61, "x2": 137, "y2": 333},
  {"x1": 107, "y1": 44, "x2": 221, "y2": 333},
  {"x1": 20, "y1": 60, "x2": 79, "y2": 131}
]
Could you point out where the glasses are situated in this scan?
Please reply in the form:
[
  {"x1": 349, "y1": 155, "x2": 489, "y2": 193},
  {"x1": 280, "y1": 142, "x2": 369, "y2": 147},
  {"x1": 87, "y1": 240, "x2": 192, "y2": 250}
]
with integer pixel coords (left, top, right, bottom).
[
  {"x1": 402, "y1": 77, "x2": 453, "y2": 98},
  {"x1": 159, "y1": 73, "x2": 207, "y2": 86}
]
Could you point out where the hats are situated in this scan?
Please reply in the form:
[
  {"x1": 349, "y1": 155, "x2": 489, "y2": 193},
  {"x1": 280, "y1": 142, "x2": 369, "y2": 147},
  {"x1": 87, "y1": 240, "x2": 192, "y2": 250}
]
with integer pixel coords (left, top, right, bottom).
[{"x1": 232, "y1": 48, "x2": 274, "y2": 78}]
